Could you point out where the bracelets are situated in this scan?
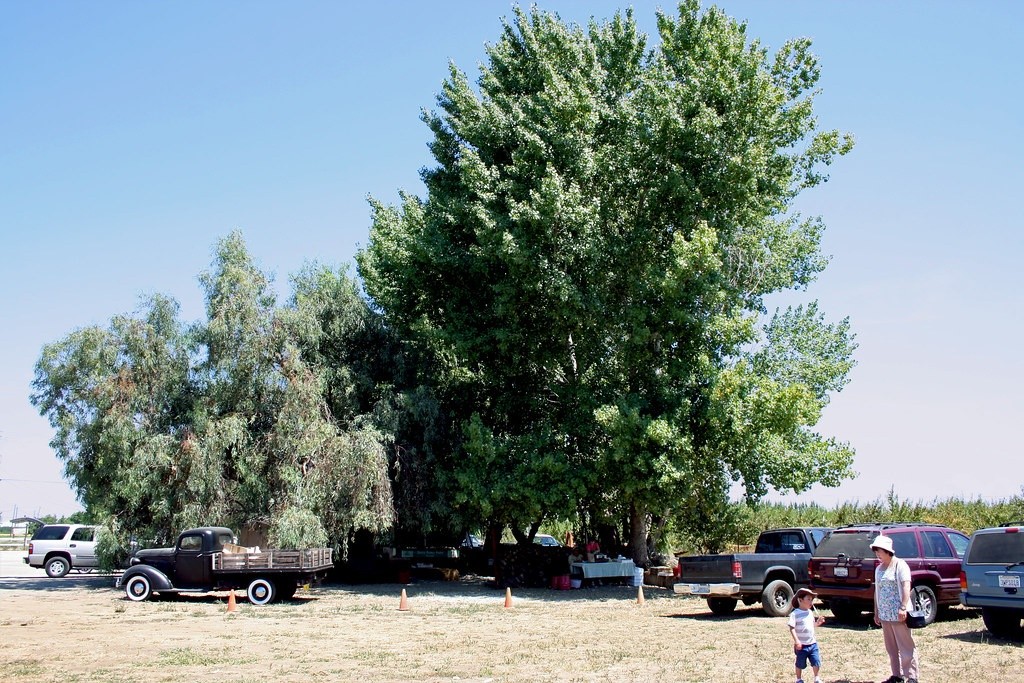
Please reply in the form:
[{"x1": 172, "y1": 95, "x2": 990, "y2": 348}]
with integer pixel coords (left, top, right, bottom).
[{"x1": 899, "y1": 605, "x2": 907, "y2": 611}]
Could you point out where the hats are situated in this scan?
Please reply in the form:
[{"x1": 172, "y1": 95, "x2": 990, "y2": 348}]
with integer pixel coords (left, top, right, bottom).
[
  {"x1": 791, "y1": 588, "x2": 818, "y2": 608},
  {"x1": 870, "y1": 535, "x2": 895, "y2": 553}
]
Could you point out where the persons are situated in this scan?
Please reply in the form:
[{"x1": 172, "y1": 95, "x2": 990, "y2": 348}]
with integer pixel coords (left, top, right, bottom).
[
  {"x1": 568, "y1": 547, "x2": 590, "y2": 589},
  {"x1": 787, "y1": 588, "x2": 826, "y2": 683},
  {"x1": 586, "y1": 537, "x2": 604, "y2": 587},
  {"x1": 869, "y1": 535, "x2": 919, "y2": 683}
]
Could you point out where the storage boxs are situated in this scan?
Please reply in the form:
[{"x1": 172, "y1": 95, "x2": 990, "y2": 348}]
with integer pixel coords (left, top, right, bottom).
[
  {"x1": 629, "y1": 566, "x2": 644, "y2": 587},
  {"x1": 588, "y1": 554, "x2": 607, "y2": 562}
]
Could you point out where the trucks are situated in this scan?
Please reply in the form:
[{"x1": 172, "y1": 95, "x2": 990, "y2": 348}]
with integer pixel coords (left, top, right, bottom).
[{"x1": 111, "y1": 525, "x2": 336, "y2": 607}]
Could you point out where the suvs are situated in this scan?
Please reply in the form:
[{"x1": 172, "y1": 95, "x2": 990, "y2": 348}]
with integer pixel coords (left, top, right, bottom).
[
  {"x1": 807, "y1": 521, "x2": 973, "y2": 627},
  {"x1": 959, "y1": 522, "x2": 1024, "y2": 639},
  {"x1": 23, "y1": 523, "x2": 141, "y2": 579}
]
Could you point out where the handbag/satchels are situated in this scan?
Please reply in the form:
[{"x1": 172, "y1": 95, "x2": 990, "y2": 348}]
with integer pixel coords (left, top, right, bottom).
[
  {"x1": 907, "y1": 610, "x2": 926, "y2": 628},
  {"x1": 588, "y1": 553, "x2": 595, "y2": 562}
]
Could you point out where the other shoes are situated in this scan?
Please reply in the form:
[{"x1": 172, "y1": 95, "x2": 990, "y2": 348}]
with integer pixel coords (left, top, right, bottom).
[
  {"x1": 881, "y1": 675, "x2": 905, "y2": 683},
  {"x1": 796, "y1": 679, "x2": 804, "y2": 683},
  {"x1": 814, "y1": 681, "x2": 819, "y2": 683},
  {"x1": 902, "y1": 675, "x2": 918, "y2": 683}
]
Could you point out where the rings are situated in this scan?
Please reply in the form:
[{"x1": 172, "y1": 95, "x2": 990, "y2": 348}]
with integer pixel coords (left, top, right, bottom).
[{"x1": 902, "y1": 617, "x2": 906, "y2": 619}]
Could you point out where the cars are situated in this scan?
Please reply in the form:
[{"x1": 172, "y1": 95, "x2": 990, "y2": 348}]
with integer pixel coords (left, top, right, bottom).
[
  {"x1": 535, "y1": 534, "x2": 560, "y2": 549},
  {"x1": 464, "y1": 536, "x2": 484, "y2": 548}
]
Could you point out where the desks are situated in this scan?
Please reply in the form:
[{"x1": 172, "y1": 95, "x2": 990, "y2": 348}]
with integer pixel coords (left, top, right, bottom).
[{"x1": 570, "y1": 560, "x2": 635, "y2": 588}]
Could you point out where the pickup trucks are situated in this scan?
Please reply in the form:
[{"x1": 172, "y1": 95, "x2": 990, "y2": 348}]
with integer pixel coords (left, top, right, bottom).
[{"x1": 672, "y1": 525, "x2": 833, "y2": 616}]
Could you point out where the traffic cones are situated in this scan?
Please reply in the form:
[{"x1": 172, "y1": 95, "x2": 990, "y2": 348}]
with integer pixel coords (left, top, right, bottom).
[
  {"x1": 503, "y1": 586, "x2": 515, "y2": 608},
  {"x1": 225, "y1": 589, "x2": 240, "y2": 613},
  {"x1": 396, "y1": 588, "x2": 412, "y2": 613},
  {"x1": 636, "y1": 585, "x2": 649, "y2": 607}
]
